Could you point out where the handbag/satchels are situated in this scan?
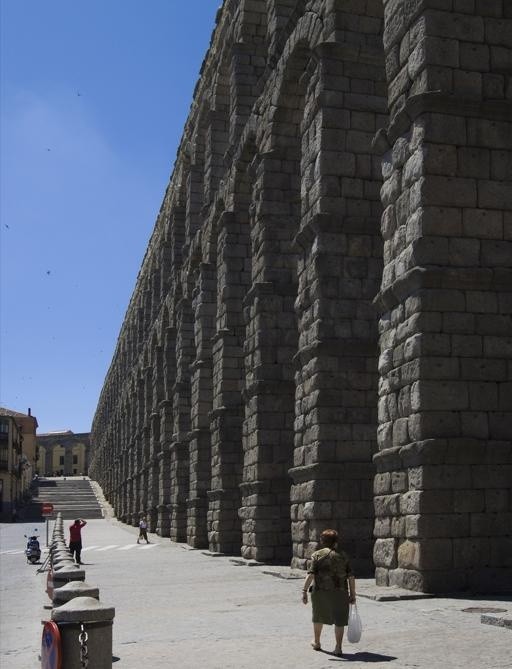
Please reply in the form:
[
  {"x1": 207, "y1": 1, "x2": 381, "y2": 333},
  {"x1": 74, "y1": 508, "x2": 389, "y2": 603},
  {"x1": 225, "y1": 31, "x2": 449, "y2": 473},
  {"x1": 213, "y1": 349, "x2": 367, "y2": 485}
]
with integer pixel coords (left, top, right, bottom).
[{"x1": 309, "y1": 585, "x2": 313, "y2": 592}]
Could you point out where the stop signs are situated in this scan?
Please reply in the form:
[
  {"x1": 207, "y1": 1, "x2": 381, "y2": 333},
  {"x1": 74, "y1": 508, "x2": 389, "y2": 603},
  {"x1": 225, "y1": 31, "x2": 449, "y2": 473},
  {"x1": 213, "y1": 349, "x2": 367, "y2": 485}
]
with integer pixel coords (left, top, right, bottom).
[{"x1": 40, "y1": 503, "x2": 53, "y2": 512}]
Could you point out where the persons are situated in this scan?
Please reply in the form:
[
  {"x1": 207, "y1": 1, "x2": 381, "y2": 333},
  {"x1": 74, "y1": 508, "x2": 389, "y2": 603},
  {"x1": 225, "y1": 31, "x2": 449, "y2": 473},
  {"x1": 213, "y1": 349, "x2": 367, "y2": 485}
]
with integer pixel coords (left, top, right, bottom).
[
  {"x1": 137, "y1": 516, "x2": 150, "y2": 544},
  {"x1": 302, "y1": 529, "x2": 356, "y2": 656},
  {"x1": 69, "y1": 518, "x2": 87, "y2": 564}
]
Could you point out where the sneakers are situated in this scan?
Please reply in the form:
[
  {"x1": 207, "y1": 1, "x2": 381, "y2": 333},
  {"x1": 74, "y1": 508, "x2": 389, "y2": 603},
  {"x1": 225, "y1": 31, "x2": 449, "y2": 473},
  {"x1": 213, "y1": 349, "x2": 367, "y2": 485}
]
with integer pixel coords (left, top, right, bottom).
[
  {"x1": 333, "y1": 649, "x2": 342, "y2": 657},
  {"x1": 311, "y1": 641, "x2": 321, "y2": 649}
]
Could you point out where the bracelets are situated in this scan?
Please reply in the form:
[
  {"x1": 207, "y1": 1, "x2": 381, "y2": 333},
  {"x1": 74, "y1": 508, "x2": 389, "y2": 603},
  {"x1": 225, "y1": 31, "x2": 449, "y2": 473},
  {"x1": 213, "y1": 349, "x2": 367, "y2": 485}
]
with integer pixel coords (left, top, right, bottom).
[{"x1": 303, "y1": 589, "x2": 307, "y2": 592}]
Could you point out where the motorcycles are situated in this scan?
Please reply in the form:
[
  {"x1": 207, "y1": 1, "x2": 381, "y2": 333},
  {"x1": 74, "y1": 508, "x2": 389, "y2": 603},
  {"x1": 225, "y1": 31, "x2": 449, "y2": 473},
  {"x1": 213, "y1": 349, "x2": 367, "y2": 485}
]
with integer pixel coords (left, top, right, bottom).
[{"x1": 23, "y1": 527, "x2": 44, "y2": 565}]
